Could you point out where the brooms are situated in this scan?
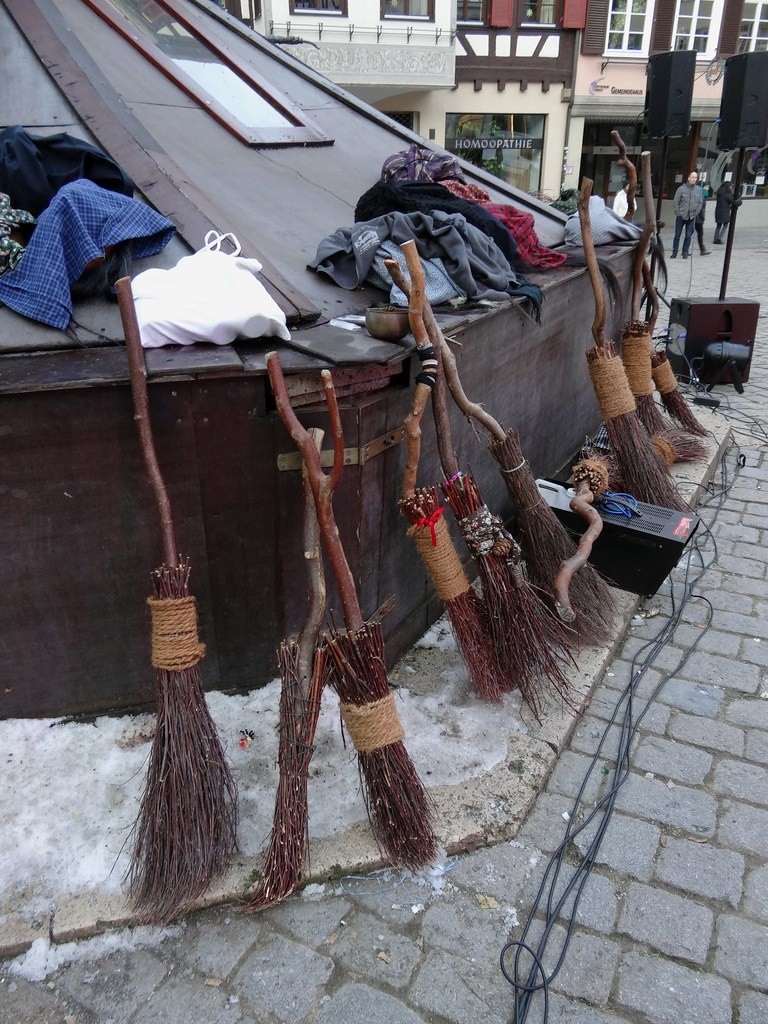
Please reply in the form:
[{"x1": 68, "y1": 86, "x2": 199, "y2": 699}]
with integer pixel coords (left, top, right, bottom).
[
  {"x1": 257, "y1": 348, "x2": 439, "y2": 912},
  {"x1": 111, "y1": 277, "x2": 246, "y2": 932},
  {"x1": 381, "y1": 130, "x2": 713, "y2": 719}
]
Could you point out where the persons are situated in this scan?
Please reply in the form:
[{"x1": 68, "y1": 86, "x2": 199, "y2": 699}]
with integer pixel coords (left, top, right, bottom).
[
  {"x1": 670, "y1": 171, "x2": 704, "y2": 259},
  {"x1": 612, "y1": 180, "x2": 638, "y2": 225},
  {"x1": 681, "y1": 182, "x2": 713, "y2": 255},
  {"x1": 713, "y1": 180, "x2": 742, "y2": 244}
]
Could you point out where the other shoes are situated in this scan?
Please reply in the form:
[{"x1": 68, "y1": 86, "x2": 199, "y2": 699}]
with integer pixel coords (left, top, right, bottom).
[
  {"x1": 701, "y1": 251, "x2": 712, "y2": 256},
  {"x1": 718, "y1": 240, "x2": 722, "y2": 244},
  {"x1": 681, "y1": 253, "x2": 691, "y2": 256},
  {"x1": 683, "y1": 255, "x2": 687, "y2": 259},
  {"x1": 670, "y1": 254, "x2": 676, "y2": 259},
  {"x1": 713, "y1": 240, "x2": 720, "y2": 243}
]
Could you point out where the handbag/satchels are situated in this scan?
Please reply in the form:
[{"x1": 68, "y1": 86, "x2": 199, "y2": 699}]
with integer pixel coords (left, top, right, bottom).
[{"x1": 130, "y1": 229, "x2": 291, "y2": 348}]
